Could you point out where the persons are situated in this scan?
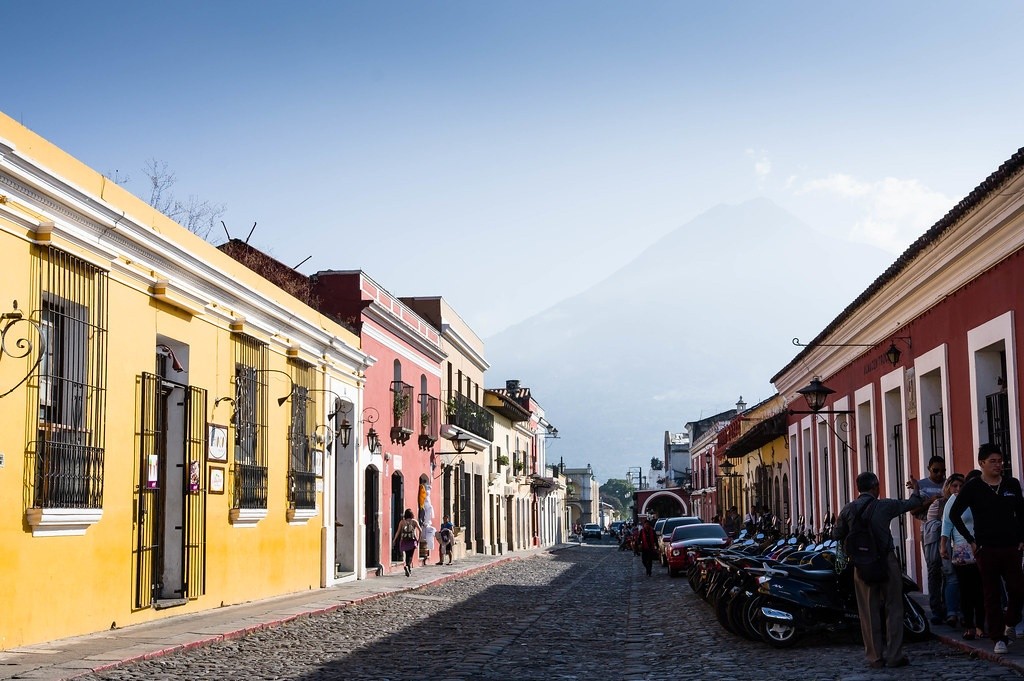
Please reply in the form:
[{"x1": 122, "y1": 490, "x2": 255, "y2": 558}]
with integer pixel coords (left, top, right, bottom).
[
  {"x1": 713, "y1": 506, "x2": 743, "y2": 535},
  {"x1": 821, "y1": 512, "x2": 833, "y2": 536},
  {"x1": 949, "y1": 442, "x2": 1024, "y2": 652},
  {"x1": 744, "y1": 506, "x2": 774, "y2": 534},
  {"x1": 637, "y1": 521, "x2": 661, "y2": 576},
  {"x1": 928, "y1": 473, "x2": 966, "y2": 627},
  {"x1": 574, "y1": 524, "x2": 583, "y2": 544},
  {"x1": 618, "y1": 523, "x2": 642, "y2": 557},
  {"x1": 436, "y1": 515, "x2": 454, "y2": 564},
  {"x1": 940, "y1": 471, "x2": 986, "y2": 640},
  {"x1": 837, "y1": 472, "x2": 923, "y2": 666},
  {"x1": 911, "y1": 455, "x2": 948, "y2": 624},
  {"x1": 393, "y1": 509, "x2": 422, "y2": 577}
]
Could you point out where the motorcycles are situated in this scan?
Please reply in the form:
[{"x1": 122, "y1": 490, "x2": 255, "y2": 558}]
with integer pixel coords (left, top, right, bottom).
[{"x1": 686, "y1": 511, "x2": 930, "y2": 648}]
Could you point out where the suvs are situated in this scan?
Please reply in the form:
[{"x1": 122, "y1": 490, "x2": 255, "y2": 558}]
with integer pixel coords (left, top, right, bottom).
[{"x1": 653, "y1": 516, "x2": 702, "y2": 564}]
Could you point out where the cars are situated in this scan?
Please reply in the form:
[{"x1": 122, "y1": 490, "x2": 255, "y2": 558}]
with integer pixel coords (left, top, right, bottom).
[
  {"x1": 583, "y1": 522, "x2": 601, "y2": 540},
  {"x1": 663, "y1": 523, "x2": 733, "y2": 576}
]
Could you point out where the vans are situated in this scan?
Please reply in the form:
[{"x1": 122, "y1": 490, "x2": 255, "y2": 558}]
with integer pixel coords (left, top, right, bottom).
[{"x1": 610, "y1": 521, "x2": 623, "y2": 536}]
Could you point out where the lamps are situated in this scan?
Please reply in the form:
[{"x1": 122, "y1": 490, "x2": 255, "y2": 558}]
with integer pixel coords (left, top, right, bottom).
[
  {"x1": 216, "y1": 396, "x2": 239, "y2": 425},
  {"x1": 307, "y1": 388, "x2": 341, "y2": 420},
  {"x1": 156, "y1": 343, "x2": 183, "y2": 372},
  {"x1": 315, "y1": 424, "x2": 333, "y2": 456},
  {"x1": 716, "y1": 456, "x2": 759, "y2": 478},
  {"x1": 787, "y1": 337, "x2": 912, "y2": 452},
  {"x1": 431, "y1": 429, "x2": 478, "y2": 479},
  {"x1": 330, "y1": 396, "x2": 353, "y2": 449},
  {"x1": 704, "y1": 444, "x2": 716, "y2": 466},
  {"x1": 358, "y1": 407, "x2": 379, "y2": 452},
  {"x1": 543, "y1": 424, "x2": 561, "y2": 448},
  {"x1": 701, "y1": 486, "x2": 710, "y2": 498},
  {"x1": 257, "y1": 369, "x2": 295, "y2": 406}
]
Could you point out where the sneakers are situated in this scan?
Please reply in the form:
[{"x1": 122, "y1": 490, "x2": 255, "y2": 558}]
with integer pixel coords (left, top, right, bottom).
[
  {"x1": 994, "y1": 641, "x2": 1009, "y2": 653},
  {"x1": 1005, "y1": 624, "x2": 1016, "y2": 644}
]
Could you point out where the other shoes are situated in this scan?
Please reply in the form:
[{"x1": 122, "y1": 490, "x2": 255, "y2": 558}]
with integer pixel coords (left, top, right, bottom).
[
  {"x1": 947, "y1": 618, "x2": 957, "y2": 627},
  {"x1": 963, "y1": 629, "x2": 975, "y2": 639},
  {"x1": 931, "y1": 613, "x2": 944, "y2": 624},
  {"x1": 446, "y1": 562, "x2": 452, "y2": 565},
  {"x1": 436, "y1": 562, "x2": 443, "y2": 565},
  {"x1": 891, "y1": 658, "x2": 909, "y2": 668},
  {"x1": 977, "y1": 631, "x2": 989, "y2": 638},
  {"x1": 404, "y1": 566, "x2": 410, "y2": 577}
]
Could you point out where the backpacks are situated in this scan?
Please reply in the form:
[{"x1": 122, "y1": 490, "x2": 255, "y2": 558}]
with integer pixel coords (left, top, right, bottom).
[
  {"x1": 843, "y1": 500, "x2": 879, "y2": 566},
  {"x1": 401, "y1": 519, "x2": 415, "y2": 539}
]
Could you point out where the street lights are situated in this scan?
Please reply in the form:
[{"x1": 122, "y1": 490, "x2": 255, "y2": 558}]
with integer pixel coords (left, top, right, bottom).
[{"x1": 626, "y1": 466, "x2": 649, "y2": 490}]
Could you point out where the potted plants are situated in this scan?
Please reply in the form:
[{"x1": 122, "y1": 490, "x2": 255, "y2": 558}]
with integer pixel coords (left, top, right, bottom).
[
  {"x1": 393, "y1": 391, "x2": 410, "y2": 427},
  {"x1": 448, "y1": 396, "x2": 493, "y2": 440},
  {"x1": 494, "y1": 455, "x2": 509, "y2": 473},
  {"x1": 514, "y1": 459, "x2": 524, "y2": 476},
  {"x1": 421, "y1": 411, "x2": 431, "y2": 435}
]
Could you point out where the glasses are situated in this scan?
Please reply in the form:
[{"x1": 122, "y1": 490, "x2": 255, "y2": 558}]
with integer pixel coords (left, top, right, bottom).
[
  {"x1": 984, "y1": 459, "x2": 1004, "y2": 465},
  {"x1": 931, "y1": 467, "x2": 947, "y2": 474}
]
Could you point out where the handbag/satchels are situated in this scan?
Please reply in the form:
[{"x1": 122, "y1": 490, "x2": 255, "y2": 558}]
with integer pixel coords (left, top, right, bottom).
[{"x1": 952, "y1": 542, "x2": 976, "y2": 565}]
